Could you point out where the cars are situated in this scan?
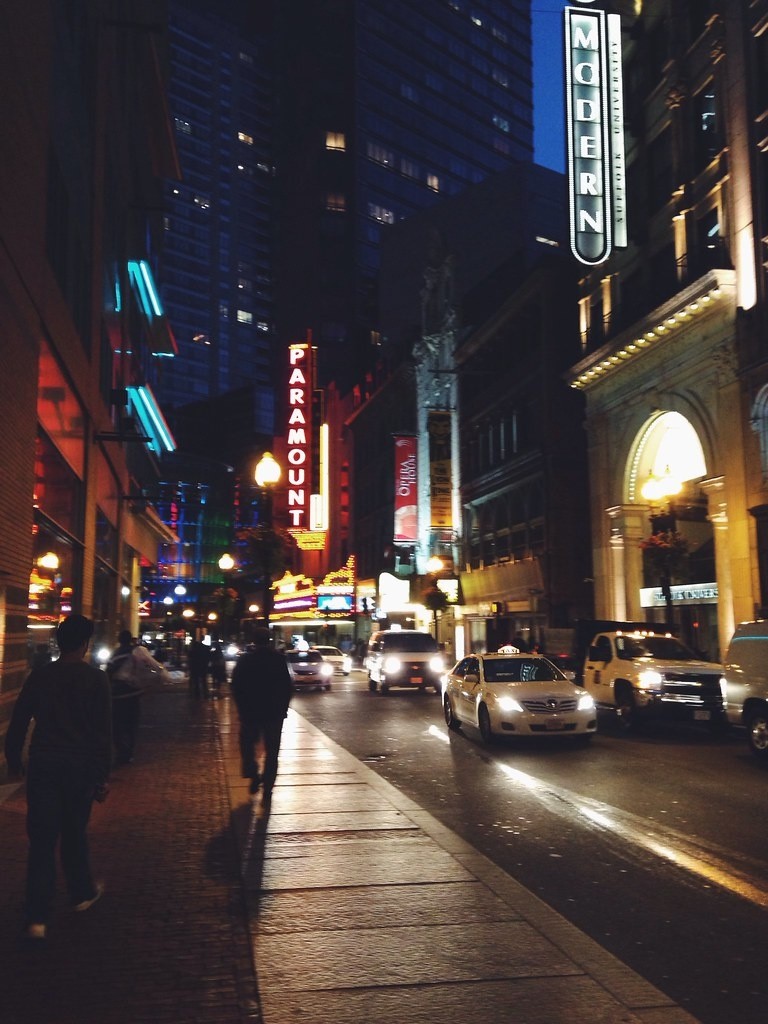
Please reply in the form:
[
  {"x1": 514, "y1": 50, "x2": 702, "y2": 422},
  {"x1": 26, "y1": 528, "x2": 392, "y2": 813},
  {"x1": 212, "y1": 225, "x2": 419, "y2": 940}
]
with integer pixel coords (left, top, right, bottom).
[
  {"x1": 441, "y1": 645, "x2": 597, "y2": 744},
  {"x1": 284, "y1": 650, "x2": 332, "y2": 691},
  {"x1": 222, "y1": 650, "x2": 241, "y2": 683},
  {"x1": 310, "y1": 646, "x2": 351, "y2": 676}
]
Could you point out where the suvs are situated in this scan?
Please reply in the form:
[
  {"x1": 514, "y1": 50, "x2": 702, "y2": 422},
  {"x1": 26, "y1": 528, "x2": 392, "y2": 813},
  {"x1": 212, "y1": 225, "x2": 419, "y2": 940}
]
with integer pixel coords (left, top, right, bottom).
[{"x1": 585, "y1": 631, "x2": 721, "y2": 727}]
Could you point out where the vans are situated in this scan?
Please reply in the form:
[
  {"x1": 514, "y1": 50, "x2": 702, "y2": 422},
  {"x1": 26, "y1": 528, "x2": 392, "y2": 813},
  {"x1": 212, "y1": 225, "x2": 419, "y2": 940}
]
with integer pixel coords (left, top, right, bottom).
[
  {"x1": 719, "y1": 619, "x2": 768, "y2": 751},
  {"x1": 368, "y1": 630, "x2": 445, "y2": 695}
]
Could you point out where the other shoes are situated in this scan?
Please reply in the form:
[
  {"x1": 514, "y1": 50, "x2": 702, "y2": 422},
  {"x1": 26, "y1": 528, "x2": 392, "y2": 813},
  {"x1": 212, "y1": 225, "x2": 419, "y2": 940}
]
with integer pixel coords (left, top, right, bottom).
[
  {"x1": 26, "y1": 924, "x2": 47, "y2": 938},
  {"x1": 250, "y1": 775, "x2": 264, "y2": 793},
  {"x1": 72, "y1": 882, "x2": 101, "y2": 912}
]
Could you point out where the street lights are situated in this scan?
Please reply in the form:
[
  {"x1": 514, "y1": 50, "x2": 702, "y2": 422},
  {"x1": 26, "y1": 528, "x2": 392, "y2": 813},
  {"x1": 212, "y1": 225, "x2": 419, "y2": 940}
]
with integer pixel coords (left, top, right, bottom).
[
  {"x1": 426, "y1": 556, "x2": 443, "y2": 642},
  {"x1": 641, "y1": 465, "x2": 682, "y2": 624},
  {"x1": 218, "y1": 553, "x2": 235, "y2": 645},
  {"x1": 255, "y1": 451, "x2": 281, "y2": 629}
]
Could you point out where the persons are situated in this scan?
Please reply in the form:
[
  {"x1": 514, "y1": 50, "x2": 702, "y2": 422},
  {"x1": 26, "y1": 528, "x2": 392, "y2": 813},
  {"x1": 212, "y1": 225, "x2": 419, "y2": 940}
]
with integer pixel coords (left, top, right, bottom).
[
  {"x1": 106, "y1": 630, "x2": 229, "y2": 761},
  {"x1": 235, "y1": 626, "x2": 293, "y2": 799},
  {"x1": 512, "y1": 630, "x2": 539, "y2": 655},
  {"x1": 3, "y1": 614, "x2": 120, "y2": 940}
]
